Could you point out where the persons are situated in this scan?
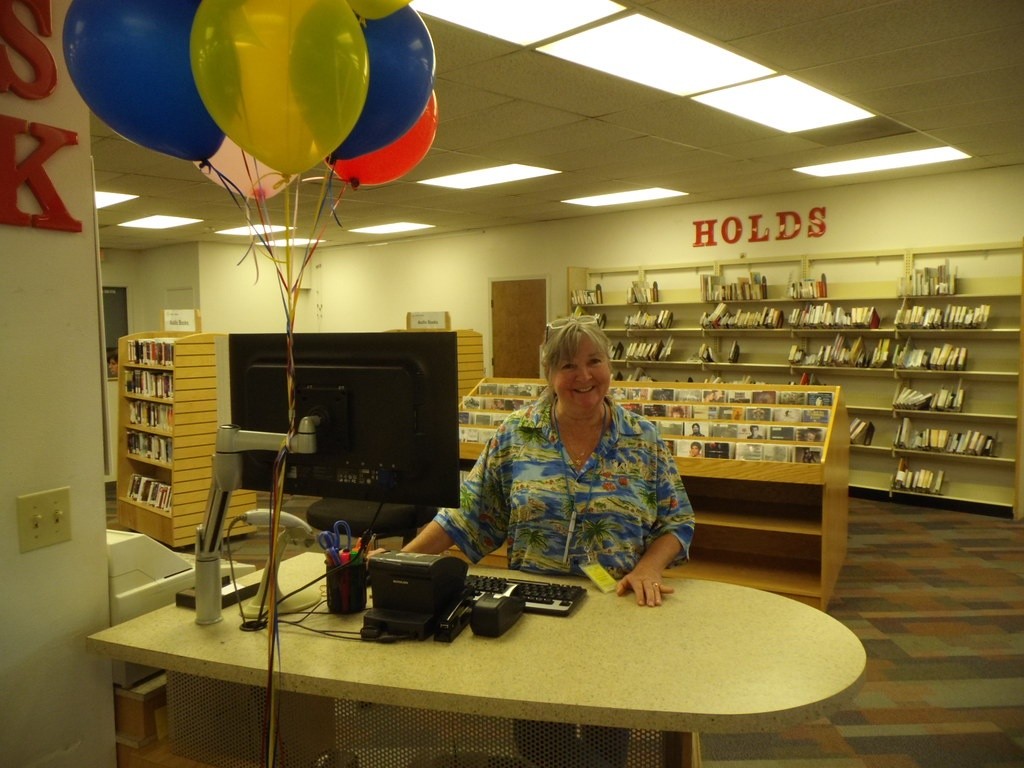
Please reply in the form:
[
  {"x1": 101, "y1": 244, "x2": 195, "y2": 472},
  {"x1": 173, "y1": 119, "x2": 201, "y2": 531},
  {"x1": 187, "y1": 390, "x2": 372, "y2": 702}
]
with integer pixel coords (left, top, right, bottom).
[
  {"x1": 107, "y1": 354, "x2": 118, "y2": 377},
  {"x1": 367, "y1": 308, "x2": 695, "y2": 768}
]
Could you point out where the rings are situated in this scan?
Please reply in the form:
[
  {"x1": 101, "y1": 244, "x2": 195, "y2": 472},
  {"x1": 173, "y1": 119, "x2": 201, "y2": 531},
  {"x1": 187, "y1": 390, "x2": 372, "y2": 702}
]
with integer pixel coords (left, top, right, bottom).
[{"x1": 653, "y1": 583, "x2": 658, "y2": 587}]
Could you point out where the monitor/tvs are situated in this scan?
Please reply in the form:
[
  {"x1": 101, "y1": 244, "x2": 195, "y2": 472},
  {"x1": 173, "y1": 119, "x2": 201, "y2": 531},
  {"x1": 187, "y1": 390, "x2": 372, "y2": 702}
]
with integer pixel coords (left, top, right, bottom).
[{"x1": 226, "y1": 332, "x2": 461, "y2": 509}]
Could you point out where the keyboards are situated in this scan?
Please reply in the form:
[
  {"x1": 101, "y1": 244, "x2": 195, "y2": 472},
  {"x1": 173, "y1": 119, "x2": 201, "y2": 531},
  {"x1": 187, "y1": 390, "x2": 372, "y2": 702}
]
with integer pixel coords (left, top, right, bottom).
[{"x1": 467, "y1": 575, "x2": 587, "y2": 616}]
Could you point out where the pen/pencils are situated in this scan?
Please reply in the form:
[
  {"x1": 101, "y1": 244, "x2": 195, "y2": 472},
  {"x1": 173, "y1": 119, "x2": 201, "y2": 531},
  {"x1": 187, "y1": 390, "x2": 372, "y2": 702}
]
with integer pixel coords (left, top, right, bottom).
[
  {"x1": 563, "y1": 511, "x2": 577, "y2": 562},
  {"x1": 355, "y1": 530, "x2": 376, "y2": 560},
  {"x1": 325, "y1": 550, "x2": 335, "y2": 564}
]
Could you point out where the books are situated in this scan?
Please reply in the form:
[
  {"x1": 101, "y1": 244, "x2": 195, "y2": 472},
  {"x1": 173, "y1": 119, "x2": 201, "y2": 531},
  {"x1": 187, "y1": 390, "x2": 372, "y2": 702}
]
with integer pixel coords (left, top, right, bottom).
[
  {"x1": 609, "y1": 336, "x2": 674, "y2": 360},
  {"x1": 892, "y1": 266, "x2": 991, "y2": 412},
  {"x1": 698, "y1": 273, "x2": 890, "y2": 367},
  {"x1": 624, "y1": 310, "x2": 672, "y2": 328},
  {"x1": 894, "y1": 417, "x2": 994, "y2": 493},
  {"x1": 571, "y1": 280, "x2": 659, "y2": 305},
  {"x1": 572, "y1": 306, "x2": 606, "y2": 329},
  {"x1": 458, "y1": 367, "x2": 834, "y2": 464},
  {"x1": 125, "y1": 337, "x2": 183, "y2": 515},
  {"x1": 849, "y1": 418, "x2": 867, "y2": 444}
]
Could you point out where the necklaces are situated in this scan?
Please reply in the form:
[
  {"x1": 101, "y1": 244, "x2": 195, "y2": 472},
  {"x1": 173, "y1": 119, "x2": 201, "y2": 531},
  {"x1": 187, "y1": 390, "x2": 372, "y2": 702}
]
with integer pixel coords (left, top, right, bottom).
[{"x1": 559, "y1": 411, "x2": 602, "y2": 467}]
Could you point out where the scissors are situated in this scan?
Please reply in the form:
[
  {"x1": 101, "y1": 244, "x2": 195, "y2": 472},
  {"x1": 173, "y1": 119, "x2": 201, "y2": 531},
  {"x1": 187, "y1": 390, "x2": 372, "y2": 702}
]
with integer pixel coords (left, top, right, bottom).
[
  {"x1": 332, "y1": 546, "x2": 339, "y2": 565},
  {"x1": 318, "y1": 521, "x2": 351, "y2": 549}
]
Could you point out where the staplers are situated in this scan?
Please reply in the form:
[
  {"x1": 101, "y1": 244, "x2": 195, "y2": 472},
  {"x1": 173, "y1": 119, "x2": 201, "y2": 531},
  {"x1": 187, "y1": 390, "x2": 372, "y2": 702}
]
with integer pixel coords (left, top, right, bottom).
[{"x1": 433, "y1": 585, "x2": 478, "y2": 644}]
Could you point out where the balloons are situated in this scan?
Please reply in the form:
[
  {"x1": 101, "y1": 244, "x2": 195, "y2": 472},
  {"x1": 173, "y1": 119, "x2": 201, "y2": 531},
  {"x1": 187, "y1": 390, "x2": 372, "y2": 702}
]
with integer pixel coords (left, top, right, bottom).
[
  {"x1": 288, "y1": 4, "x2": 436, "y2": 165},
  {"x1": 192, "y1": 135, "x2": 299, "y2": 201},
  {"x1": 189, "y1": 0, "x2": 369, "y2": 183},
  {"x1": 63, "y1": 0, "x2": 226, "y2": 173},
  {"x1": 324, "y1": 88, "x2": 438, "y2": 188},
  {"x1": 345, "y1": 0, "x2": 413, "y2": 27}
]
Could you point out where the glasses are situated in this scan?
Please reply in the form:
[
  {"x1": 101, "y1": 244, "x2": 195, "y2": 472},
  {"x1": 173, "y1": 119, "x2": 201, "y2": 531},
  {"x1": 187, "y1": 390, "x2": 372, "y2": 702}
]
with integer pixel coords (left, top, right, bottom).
[{"x1": 546, "y1": 316, "x2": 600, "y2": 344}]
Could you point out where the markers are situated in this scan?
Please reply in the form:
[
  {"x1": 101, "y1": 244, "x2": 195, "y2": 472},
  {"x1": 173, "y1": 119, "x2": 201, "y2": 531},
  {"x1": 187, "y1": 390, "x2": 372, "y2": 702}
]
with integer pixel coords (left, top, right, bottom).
[{"x1": 339, "y1": 548, "x2": 363, "y2": 563}]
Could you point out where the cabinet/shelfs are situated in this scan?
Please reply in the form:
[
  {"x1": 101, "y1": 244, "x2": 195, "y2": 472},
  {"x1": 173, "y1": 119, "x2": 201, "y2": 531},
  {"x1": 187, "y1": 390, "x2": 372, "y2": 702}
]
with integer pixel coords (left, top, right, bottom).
[
  {"x1": 115, "y1": 332, "x2": 257, "y2": 546},
  {"x1": 460, "y1": 374, "x2": 849, "y2": 615},
  {"x1": 568, "y1": 291, "x2": 1024, "y2": 512}
]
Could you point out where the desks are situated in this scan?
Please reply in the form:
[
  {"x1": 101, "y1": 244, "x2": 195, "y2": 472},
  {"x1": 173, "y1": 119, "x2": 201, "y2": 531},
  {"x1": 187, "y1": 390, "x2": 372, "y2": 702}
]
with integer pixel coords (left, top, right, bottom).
[{"x1": 84, "y1": 553, "x2": 867, "y2": 768}]
